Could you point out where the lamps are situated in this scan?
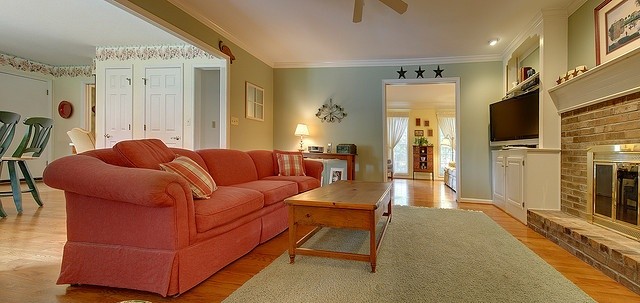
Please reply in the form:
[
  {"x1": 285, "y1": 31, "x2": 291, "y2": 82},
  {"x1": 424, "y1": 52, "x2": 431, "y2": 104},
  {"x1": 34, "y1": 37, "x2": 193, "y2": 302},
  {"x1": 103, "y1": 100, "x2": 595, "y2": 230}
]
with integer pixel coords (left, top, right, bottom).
[{"x1": 294, "y1": 123, "x2": 309, "y2": 152}]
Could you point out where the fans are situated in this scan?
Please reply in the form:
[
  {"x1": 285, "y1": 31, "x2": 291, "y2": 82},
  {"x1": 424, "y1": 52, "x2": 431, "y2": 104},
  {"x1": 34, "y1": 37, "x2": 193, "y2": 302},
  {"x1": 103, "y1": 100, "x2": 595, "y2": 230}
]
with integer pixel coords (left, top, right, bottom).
[{"x1": 353, "y1": 0, "x2": 409, "y2": 23}]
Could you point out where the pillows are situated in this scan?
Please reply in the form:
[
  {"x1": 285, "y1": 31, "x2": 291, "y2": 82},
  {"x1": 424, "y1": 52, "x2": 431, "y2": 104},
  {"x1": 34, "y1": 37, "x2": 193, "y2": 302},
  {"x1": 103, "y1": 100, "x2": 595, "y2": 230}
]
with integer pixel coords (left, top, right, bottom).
[
  {"x1": 112, "y1": 139, "x2": 176, "y2": 171},
  {"x1": 277, "y1": 153, "x2": 306, "y2": 178},
  {"x1": 273, "y1": 149, "x2": 305, "y2": 176},
  {"x1": 159, "y1": 153, "x2": 218, "y2": 199}
]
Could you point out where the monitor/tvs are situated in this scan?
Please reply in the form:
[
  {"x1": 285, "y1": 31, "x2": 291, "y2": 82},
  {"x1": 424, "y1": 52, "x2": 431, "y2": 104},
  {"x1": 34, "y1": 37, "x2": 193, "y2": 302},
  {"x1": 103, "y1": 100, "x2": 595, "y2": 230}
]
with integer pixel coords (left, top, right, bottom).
[{"x1": 489, "y1": 88, "x2": 538, "y2": 150}]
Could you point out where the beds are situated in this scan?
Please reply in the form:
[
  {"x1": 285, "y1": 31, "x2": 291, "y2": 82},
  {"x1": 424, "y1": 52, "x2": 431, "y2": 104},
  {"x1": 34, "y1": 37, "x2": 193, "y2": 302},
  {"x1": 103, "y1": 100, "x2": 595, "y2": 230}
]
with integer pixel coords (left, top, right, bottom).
[{"x1": 444, "y1": 166, "x2": 457, "y2": 192}]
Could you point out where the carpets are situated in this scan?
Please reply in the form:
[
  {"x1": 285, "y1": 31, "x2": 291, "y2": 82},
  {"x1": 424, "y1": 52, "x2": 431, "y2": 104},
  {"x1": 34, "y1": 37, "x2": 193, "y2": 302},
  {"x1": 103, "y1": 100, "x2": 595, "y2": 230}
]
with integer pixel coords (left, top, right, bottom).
[{"x1": 219, "y1": 204, "x2": 598, "y2": 302}]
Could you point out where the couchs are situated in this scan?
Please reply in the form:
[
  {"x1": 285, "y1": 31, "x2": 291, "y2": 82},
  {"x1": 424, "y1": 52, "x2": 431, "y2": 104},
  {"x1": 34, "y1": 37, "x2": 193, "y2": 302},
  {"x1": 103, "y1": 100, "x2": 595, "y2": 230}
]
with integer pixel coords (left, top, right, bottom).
[{"x1": 43, "y1": 138, "x2": 324, "y2": 296}]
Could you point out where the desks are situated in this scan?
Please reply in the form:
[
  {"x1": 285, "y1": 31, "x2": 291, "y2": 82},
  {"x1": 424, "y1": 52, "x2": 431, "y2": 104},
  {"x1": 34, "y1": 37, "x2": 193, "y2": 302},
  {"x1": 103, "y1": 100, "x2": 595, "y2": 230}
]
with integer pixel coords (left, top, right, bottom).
[{"x1": 304, "y1": 153, "x2": 357, "y2": 180}]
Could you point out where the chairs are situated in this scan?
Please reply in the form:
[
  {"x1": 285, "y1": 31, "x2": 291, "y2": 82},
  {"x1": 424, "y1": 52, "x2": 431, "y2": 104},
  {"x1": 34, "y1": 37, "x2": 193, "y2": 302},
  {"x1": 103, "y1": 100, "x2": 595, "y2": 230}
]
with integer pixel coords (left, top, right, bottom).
[
  {"x1": 67, "y1": 128, "x2": 95, "y2": 155},
  {"x1": 0, "y1": 110, "x2": 21, "y2": 218},
  {"x1": 2, "y1": 118, "x2": 54, "y2": 213}
]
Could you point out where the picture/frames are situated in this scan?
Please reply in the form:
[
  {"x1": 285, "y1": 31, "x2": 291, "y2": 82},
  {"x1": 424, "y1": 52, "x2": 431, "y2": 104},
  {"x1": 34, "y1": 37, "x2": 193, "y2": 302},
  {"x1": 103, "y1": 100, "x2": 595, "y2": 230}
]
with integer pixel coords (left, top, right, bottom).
[
  {"x1": 245, "y1": 81, "x2": 265, "y2": 121},
  {"x1": 593, "y1": 0, "x2": 639, "y2": 67},
  {"x1": 416, "y1": 118, "x2": 420, "y2": 126},
  {"x1": 414, "y1": 130, "x2": 424, "y2": 136},
  {"x1": 425, "y1": 121, "x2": 429, "y2": 126},
  {"x1": 428, "y1": 130, "x2": 433, "y2": 137}
]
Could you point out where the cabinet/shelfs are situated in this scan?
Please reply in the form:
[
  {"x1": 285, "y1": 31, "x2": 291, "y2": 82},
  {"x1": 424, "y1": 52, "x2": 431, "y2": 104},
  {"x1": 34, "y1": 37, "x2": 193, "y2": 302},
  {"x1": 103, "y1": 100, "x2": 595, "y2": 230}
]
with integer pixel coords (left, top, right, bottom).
[
  {"x1": 413, "y1": 145, "x2": 433, "y2": 180},
  {"x1": 492, "y1": 147, "x2": 562, "y2": 225}
]
